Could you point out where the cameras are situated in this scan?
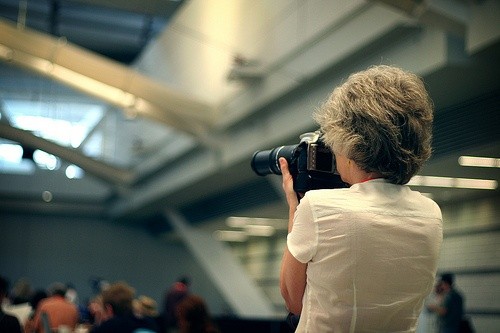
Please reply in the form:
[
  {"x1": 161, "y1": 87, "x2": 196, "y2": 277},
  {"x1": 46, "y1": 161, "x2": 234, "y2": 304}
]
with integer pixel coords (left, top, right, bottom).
[{"x1": 251, "y1": 132, "x2": 346, "y2": 193}]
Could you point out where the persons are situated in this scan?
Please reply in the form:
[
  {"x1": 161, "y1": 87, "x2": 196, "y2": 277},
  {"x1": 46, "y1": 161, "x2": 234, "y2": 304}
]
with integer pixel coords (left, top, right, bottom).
[
  {"x1": 279, "y1": 65, "x2": 444, "y2": 333},
  {"x1": 162, "y1": 276, "x2": 213, "y2": 332},
  {"x1": 426, "y1": 271, "x2": 478, "y2": 333},
  {"x1": 1, "y1": 277, "x2": 158, "y2": 333}
]
